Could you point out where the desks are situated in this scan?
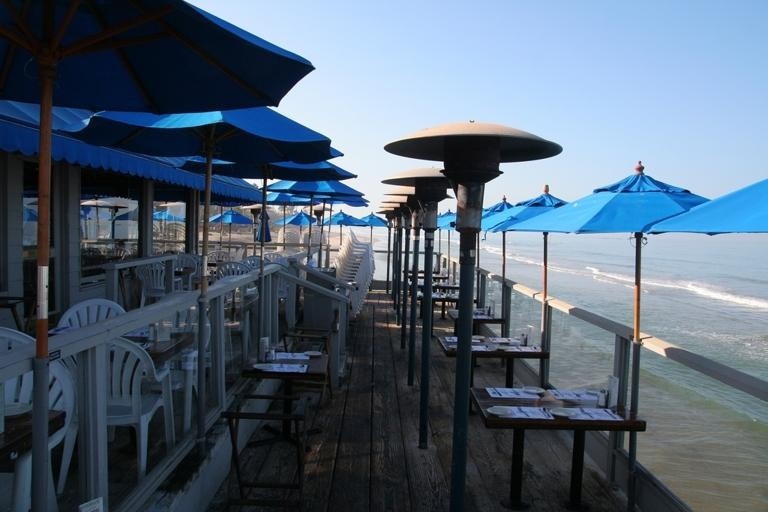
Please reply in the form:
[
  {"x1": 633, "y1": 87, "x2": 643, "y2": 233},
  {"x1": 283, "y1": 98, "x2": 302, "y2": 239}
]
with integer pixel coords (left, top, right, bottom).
[
  {"x1": 401, "y1": 265, "x2": 509, "y2": 367},
  {"x1": 436, "y1": 334, "x2": 550, "y2": 409},
  {"x1": 467, "y1": 384, "x2": 647, "y2": 511}
]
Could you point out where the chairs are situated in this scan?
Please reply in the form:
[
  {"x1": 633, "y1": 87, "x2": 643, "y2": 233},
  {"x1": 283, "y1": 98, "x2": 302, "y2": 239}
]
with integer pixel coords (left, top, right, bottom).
[
  {"x1": 328, "y1": 227, "x2": 376, "y2": 317},
  {"x1": 1, "y1": 247, "x2": 294, "y2": 512}
]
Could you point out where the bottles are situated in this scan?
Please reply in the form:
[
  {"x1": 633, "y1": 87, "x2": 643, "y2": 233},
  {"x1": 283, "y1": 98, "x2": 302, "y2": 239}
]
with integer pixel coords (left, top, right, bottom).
[
  {"x1": 598, "y1": 388, "x2": 606, "y2": 407},
  {"x1": 259, "y1": 337, "x2": 269, "y2": 363},
  {"x1": 520, "y1": 334, "x2": 526, "y2": 346}
]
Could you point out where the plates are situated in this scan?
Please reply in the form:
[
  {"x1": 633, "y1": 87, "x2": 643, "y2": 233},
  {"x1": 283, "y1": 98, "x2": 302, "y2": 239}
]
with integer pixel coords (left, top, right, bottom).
[
  {"x1": 5, "y1": 402, "x2": 31, "y2": 418},
  {"x1": 472, "y1": 335, "x2": 485, "y2": 340},
  {"x1": 549, "y1": 408, "x2": 577, "y2": 417},
  {"x1": 304, "y1": 351, "x2": 322, "y2": 356},
  {"x1": 487, "y1": 406, "x2": 513, "y2": 417},
  {"x1": 523, "y1": 385, "x2": 545, "y2": 394}
]
